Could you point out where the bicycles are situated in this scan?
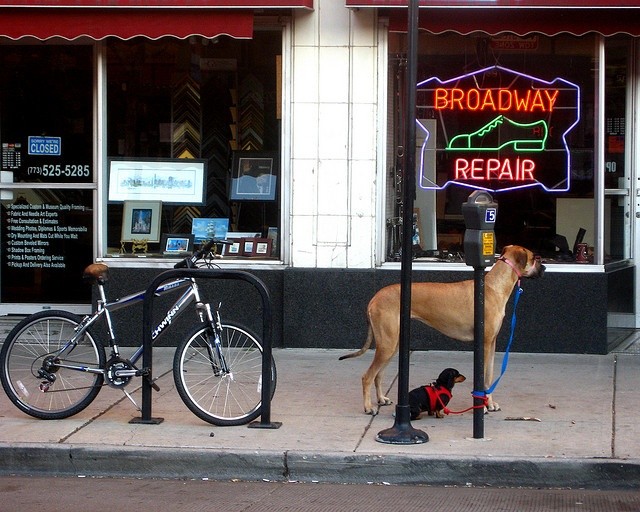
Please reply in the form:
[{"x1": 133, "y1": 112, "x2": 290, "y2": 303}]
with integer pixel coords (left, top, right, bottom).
[{"x1": 0, "y1": 236, "x2": 277, "y2": 426}]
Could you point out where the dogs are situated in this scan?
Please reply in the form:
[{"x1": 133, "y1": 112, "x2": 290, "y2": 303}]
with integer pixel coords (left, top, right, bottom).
[
  {"x1": 339, "y1": 244, "x2": 546, "y2": 415},
  {"x1": 409, "y1": 368, "x2": 466, "y2": 419}
]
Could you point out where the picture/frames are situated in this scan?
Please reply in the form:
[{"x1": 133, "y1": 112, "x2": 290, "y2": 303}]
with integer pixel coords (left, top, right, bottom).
[
  {"x1": 224, "y1": 237, "x2": 244, "y2": 256},
  {"x1": 229, "y1": 148, "x2": 277, "y2": 201},
  {"x1": 243, "y1": 237, "x2": 253, "y2": 255},
  {"x1": 104, "y1": 156, "x2": 208, "y2": 208},
  {"x1": 250, "y1": 238, "x2": 272, "y2": 257},
  {"x1": 159, "y1": 232, "x2": 194, "y2": 257},
  {"x1": 119, "y1": 196, "x2": 162, "y2": 243}
]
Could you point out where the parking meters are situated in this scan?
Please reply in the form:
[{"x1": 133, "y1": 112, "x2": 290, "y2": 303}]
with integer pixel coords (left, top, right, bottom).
[{"x1": 462, "y1": 189, "x2": 498, "y2": 438}]
